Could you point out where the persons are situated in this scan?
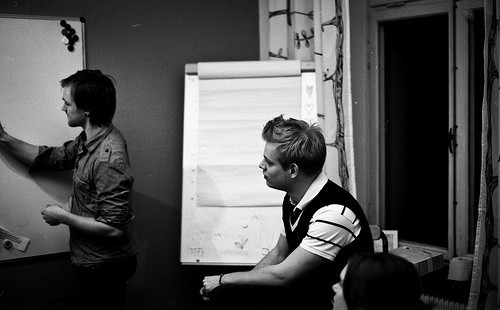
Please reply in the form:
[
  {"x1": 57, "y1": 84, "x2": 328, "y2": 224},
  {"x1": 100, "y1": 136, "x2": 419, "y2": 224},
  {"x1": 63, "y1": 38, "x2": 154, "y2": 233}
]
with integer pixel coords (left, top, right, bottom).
[
  {"x1": 1, "y1": 71, "x2": 137, "y2": 309},
  {"x1": 331, "y1": 253, "x2": 419, "y2": 310},
  {"x1": 200, "y1": 114, "x2": 374, "y2": 310}
]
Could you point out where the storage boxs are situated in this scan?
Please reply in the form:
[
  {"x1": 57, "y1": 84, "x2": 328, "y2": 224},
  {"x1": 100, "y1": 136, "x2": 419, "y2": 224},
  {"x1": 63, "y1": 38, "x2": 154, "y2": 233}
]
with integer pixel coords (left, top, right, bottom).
[{"x1": 387, "y1": 245, "x2": 445, "y2": 277}]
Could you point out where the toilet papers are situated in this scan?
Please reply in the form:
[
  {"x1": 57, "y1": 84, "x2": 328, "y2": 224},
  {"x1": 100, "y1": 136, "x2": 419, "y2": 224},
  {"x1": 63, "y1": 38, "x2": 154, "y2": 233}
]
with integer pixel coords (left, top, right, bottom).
[{"x1": 448, "y1": 256, "x2": 473, "y2": 281}]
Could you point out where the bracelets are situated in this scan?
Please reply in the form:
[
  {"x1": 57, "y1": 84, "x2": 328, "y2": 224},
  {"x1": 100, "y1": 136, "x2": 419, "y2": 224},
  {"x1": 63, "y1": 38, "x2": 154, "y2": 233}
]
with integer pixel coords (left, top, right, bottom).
[{"x1": 219, "y1": 274, "x2": 223, "y2": 286}]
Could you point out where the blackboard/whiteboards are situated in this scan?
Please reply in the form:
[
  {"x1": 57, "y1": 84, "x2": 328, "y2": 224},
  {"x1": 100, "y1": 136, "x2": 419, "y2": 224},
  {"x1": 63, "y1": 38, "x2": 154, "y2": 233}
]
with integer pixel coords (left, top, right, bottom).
[{"x1": 0, "y1": 18, "x2": 84, "y2": 260}]
